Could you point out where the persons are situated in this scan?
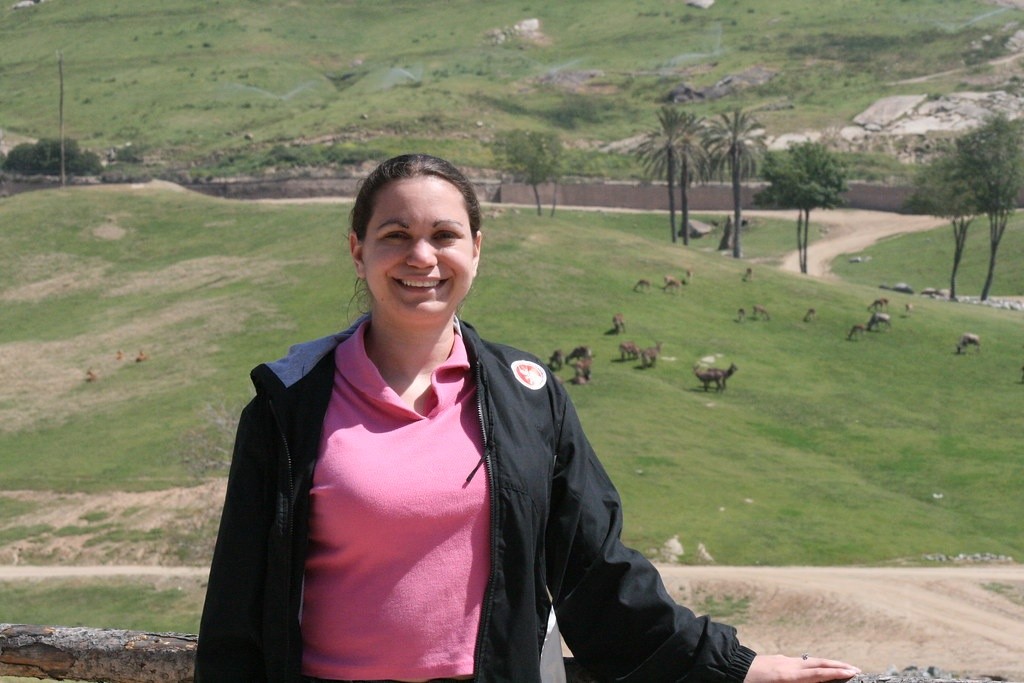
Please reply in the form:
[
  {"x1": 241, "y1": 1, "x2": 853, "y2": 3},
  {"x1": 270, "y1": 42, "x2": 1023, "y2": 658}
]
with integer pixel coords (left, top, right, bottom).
[{"x1": 193, "y1": 154, "x2": 861, "y2": 683}]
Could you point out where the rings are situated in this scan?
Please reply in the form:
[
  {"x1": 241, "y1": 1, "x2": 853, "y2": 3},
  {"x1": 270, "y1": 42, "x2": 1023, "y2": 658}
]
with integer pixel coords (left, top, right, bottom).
[{"x1": 802, "y1": 653, "x2": 808, "y2": 660}]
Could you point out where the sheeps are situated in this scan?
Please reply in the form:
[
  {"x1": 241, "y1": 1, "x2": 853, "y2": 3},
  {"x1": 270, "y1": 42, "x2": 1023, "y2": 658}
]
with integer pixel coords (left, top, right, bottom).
[
  {"x1": 846, "y1": 297, "x2": 912, "y2": 340},
  {"x1": 549, "y1": 305, "x2": 770, "y2": 393},
  {"x1": 803, "y1": 307, "x2": 816, "y2": 321},
  {"x1": 955, "y1": 334, "x2": 979, "y2": 353},
  {"x1": 633, "y1": 269, "x2": 693, "y2": 293},
  {"x1": 87, "y1": 350, "x2": 144, "y2": 383},
  {"x1": 742, "y1": 267, "x2": 752, "y2": 281}
]
